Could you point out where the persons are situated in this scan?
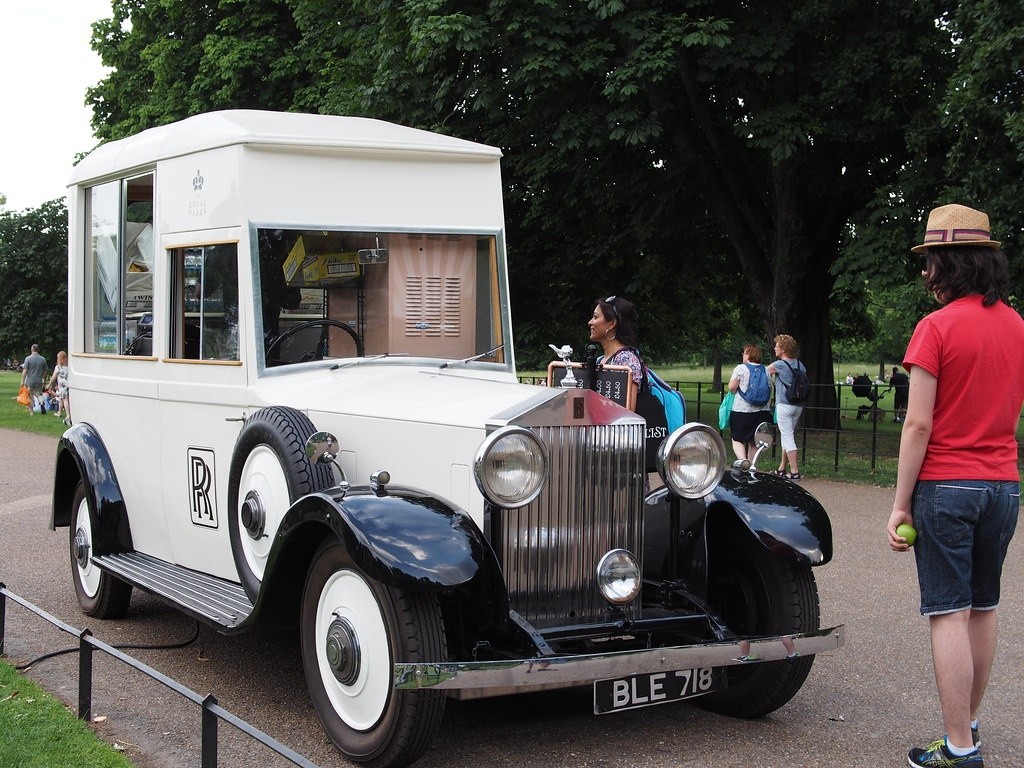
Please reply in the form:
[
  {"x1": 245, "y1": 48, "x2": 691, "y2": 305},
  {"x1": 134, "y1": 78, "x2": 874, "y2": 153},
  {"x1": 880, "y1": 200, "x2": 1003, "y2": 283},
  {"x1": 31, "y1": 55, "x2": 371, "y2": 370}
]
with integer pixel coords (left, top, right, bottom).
[
  {"x1": 3, "y1": 343, "x2": 72, "y2": 425},
  {"x1": 846, "y1": 369, "x2": 892, "y2": 386},
  {"x1": 728, "y1": 343, "x2": 774, "y2": 467},
  {"x1": 194, "y1": 229, "x2": 302, "y2": 363},
  {"x1": 887, "y1": 204, "x2": 1024, "y2": 768},
  {"x1": 767, "y1": 334, "x2": 807, "y2": 481},
  {"x1": 588, "y1": 296, "x2": 651, "y2": 495},
  {"x1": 890, "y1": 367, "x2": 909, "y2": 424}
]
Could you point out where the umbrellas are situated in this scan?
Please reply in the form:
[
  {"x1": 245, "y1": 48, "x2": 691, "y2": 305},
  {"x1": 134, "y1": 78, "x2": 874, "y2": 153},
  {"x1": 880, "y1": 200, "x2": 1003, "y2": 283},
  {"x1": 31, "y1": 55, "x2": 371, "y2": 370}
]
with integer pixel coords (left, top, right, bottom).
[{"x1": 852, "y1": 375, "x2": 872, "y2": 397}]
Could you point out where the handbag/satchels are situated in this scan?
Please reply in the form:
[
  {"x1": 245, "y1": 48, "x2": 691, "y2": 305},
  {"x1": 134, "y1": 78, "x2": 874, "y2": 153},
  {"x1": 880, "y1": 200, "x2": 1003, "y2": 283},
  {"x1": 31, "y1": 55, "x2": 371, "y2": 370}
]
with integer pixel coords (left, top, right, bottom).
[
  {"x1": 271, "y1": 250, "x2": 302, "y2": 310},
  {"x1": 17, "y1": 385, "x2": 31, "y2": 405},
  {"x1": 645, "y1": 366, "x2": 686, "y2": 436},
  {"x1": 718, "y1": 391, "x2": 735, "y2": 430},
  {"x1": 605, "y1": 346, "x2": 669, "y2": 474}
]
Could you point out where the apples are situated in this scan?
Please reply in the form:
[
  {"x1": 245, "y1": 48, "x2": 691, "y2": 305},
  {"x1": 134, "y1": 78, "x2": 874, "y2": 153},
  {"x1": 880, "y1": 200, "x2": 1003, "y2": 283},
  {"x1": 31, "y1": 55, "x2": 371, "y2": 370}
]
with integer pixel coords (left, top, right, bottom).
[{"x1": 895, "y1": 523, "x2": 917, "y2": 547}]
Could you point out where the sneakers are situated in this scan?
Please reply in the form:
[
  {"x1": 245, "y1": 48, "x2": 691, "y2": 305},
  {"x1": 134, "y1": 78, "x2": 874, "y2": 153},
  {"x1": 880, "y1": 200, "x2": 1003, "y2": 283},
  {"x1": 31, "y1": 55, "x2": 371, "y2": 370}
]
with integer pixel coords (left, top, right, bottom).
[
  {"x1": 971, "y1": 725, "x2": 982, "y2": 748},
  {"x1": 908, "y1": 734, "x2": 984, "y2": 768}
]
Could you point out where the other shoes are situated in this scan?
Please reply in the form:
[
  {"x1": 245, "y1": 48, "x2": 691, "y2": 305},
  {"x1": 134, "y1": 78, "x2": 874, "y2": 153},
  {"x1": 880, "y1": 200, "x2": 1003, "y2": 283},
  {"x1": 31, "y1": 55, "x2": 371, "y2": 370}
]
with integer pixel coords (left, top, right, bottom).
[
  {"x1": 29, "y1": 410, "x2": 34, "y2": 415},
  {"x1": 41, "y1": 404, "x2": 46, "y2": 414},
  {"x1": 53, "y1": 413, "x2": 62, "y2": 417}
]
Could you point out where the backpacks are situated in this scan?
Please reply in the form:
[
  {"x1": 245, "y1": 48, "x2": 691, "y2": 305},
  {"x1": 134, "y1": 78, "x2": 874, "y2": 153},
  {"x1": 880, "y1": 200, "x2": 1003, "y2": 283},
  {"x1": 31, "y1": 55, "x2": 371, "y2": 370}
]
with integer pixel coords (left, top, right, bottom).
[
  {"x1": 777, "y1": 359, "x2": 810, "y2": 403},
  {"x1": 737, "y1": 363, "x2": 771, "y2": 407}
]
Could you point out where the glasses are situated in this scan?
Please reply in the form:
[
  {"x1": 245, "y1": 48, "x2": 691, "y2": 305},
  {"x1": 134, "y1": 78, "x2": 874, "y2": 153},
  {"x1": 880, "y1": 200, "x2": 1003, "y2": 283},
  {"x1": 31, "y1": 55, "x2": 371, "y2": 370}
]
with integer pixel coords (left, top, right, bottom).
[{"x1": 605, "y1": 296, "x2": 617, "y2": 316}]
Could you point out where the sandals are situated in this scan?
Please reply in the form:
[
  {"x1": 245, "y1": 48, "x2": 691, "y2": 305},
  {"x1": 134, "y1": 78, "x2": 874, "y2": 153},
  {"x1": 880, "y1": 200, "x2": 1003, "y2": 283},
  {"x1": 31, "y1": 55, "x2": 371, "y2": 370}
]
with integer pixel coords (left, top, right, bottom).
[
  {"x1": 783, "y1": 472, "x2": 800, "y2": 481},
  {"x1": 769, "y1": 468, "x2": 786, "y2": 476}
]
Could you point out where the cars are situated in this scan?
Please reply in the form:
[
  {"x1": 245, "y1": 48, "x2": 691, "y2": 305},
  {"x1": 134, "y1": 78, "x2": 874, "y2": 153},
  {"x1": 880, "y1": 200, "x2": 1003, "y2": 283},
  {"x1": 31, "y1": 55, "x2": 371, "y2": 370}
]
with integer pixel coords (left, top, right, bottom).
[{"x1": 49, "y1": 109, "x2": 848, "y2": 768}]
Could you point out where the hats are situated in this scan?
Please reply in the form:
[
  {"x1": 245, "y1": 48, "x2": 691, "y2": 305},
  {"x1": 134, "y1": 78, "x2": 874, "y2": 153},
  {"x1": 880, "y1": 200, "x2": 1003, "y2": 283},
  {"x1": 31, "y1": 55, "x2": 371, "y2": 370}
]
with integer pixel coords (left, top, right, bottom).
[{"x1": 911, "y1": 204, "x2": 1002, "y2": 253}]
